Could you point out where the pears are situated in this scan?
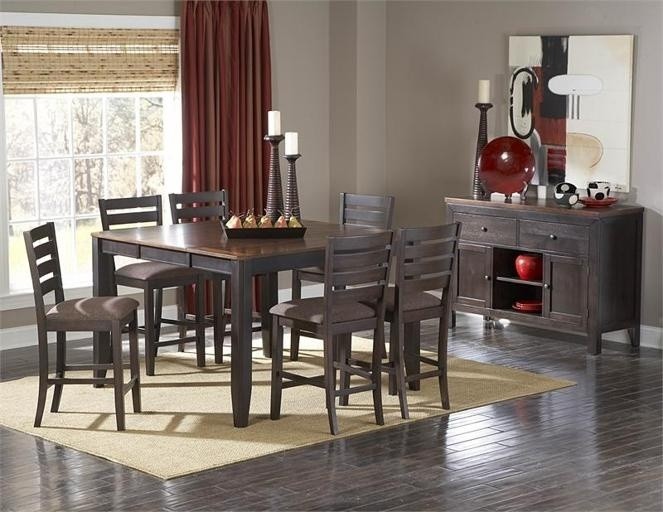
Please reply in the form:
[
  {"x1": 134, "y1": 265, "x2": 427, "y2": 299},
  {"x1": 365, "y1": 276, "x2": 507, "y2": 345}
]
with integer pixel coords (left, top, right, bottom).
[{"x1": 225, "y1": 208, "x2": 302, "y2": 228}]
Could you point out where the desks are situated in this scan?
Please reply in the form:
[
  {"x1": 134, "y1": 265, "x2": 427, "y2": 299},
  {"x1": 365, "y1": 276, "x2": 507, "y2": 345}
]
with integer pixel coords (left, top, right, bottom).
[{"x1": 90, "y1": 219, "x2": 420, "y2": 427}]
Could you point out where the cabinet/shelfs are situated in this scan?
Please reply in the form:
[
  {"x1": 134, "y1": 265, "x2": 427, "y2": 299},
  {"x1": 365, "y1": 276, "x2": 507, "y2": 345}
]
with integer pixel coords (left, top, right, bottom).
[{"x1": 444, "y1": 196, "x2": 644, "y2": 355}]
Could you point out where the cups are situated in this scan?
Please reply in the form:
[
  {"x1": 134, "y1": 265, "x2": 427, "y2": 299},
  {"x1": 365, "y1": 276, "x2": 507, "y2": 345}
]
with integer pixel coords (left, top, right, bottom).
[
  {"x1": 489, "y1": 193, "x2": 505, "y2": 202},
  {"x1": 512, "y1": 192, "x2": 520, "y2": 204},
  {"x1": 537, "y1": 185, "x2": 546, "y2": 199}
]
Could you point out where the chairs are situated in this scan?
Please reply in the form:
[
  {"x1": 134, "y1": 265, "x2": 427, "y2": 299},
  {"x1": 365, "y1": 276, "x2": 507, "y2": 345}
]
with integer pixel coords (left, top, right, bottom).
[
  {"x1": 23, "y1": 222, "x2": 140, "y2": 431},
  {"x1": 168, "y1": 189, "x2": 272, "y2": 364},
  {"x1": 291, "y1": 193, "x2": 396, "y2": 370},
  {"x1": 268, "y1": 229, "x2": 395, "y2": 436},
  {"x1": 98, "y1": 194, "x2": 205, "y2": 375},
  {"x1": 343, "y1": 220, "x2": 463, "y2": 419}
]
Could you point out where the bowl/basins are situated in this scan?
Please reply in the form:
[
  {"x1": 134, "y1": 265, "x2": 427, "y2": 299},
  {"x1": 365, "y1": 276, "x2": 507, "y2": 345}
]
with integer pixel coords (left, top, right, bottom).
[
  {"x1": 554, "y1": 193, "x2": 579, "y2": 208},
  {"x1": 587, "y1": 188, "x2": 610, "y2": 200}
]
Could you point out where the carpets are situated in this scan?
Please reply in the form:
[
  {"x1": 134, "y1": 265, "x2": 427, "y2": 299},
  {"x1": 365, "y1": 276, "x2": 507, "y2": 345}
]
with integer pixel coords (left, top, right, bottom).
[{"x1": 0, "y1": 332, "x2": 578, "y2": 482}]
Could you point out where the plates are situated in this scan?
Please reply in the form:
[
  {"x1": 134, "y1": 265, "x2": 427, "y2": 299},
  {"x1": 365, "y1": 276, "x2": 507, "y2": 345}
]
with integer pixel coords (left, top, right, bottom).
[
  {"x1": 477, "y1": 136, "x2": 536, "y2": 194},
  {"x1": 579, "y1": 197, "x2": 617, "y2": 208},
  {"x1": 511, "y1": 300, "x2": 542, "y2": 312}
]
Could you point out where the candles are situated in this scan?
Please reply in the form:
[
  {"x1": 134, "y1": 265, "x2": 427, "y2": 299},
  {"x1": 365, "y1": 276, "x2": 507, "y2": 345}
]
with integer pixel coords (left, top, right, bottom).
[
  {"x1": 268, "y1": 110, "x2": 280, "y2": 136},
  {"x1": 285, "y1": 131, "x2": 298, "y2": 155},
  {"x1": 478, "y1": 79, "x2": 490, "y2": 103}
]
